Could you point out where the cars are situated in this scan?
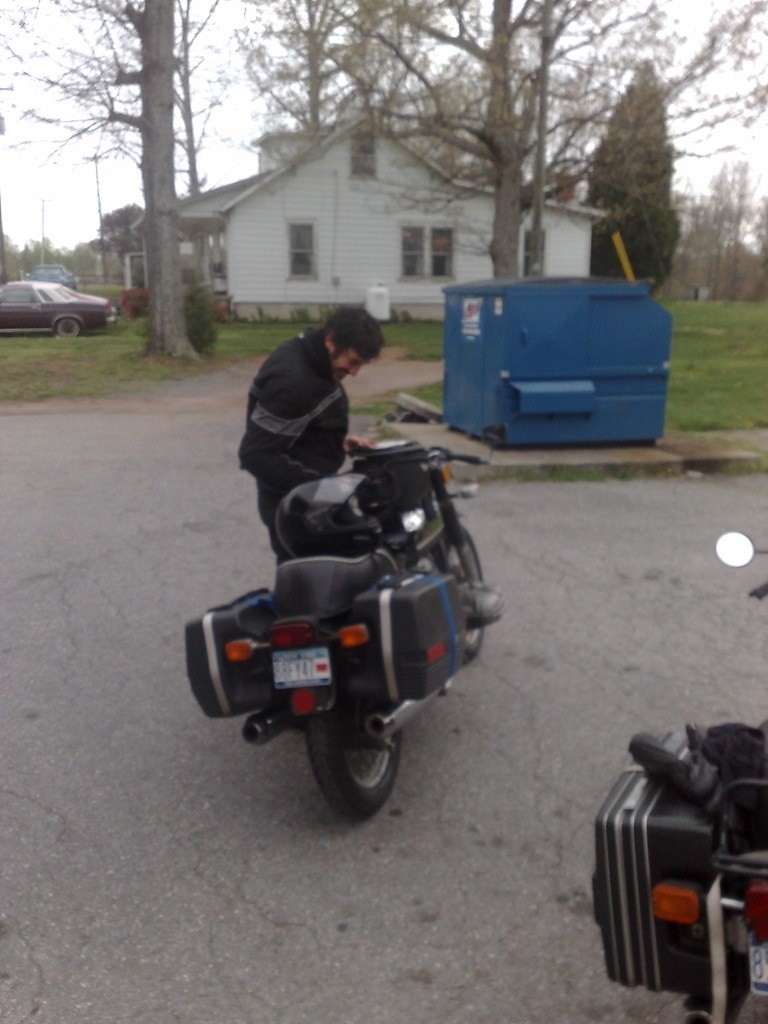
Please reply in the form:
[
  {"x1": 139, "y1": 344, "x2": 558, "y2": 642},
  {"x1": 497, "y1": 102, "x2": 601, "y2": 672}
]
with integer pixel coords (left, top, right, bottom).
[
  {"x1": 26, "y1": 265, "x2": 76, "y2": 290},
  {"x1": 0, "y1": 281, "x2": 112, "y2": 338}
]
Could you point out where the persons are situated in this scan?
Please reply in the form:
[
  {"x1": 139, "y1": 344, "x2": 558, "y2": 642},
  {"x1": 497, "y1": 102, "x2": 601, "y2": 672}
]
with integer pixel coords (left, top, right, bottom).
[{"x1": 237, "y1": 308, "x2": 385, "y2": 564}]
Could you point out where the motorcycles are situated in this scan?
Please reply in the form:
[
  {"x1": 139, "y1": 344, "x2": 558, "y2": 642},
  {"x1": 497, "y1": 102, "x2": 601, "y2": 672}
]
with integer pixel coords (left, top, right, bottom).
[
  {"x1": 184, "y1": 439, "x2": 504, "y2": 818},
  {"x1": 592, "y1": 532, "x2": 768, "y2": 1024}
]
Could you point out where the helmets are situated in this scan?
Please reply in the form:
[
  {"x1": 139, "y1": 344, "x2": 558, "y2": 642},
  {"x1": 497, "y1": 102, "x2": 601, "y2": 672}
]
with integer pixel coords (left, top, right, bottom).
[{"x1": 275, "y1": 474, "x2": 382, "y2": 559}]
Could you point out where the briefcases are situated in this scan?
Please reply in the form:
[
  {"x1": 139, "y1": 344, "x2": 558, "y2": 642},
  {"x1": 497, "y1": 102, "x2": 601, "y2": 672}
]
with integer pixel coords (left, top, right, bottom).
[
  {"x1": 185, "y1": 588, "x2": 275, "y2": 719},
  {"x1": 354, "y1": 574, "x2": 466, "y2": 704},
  {"x1": 592, "y1": 727, "x2": 726, "y2": 992}
]
